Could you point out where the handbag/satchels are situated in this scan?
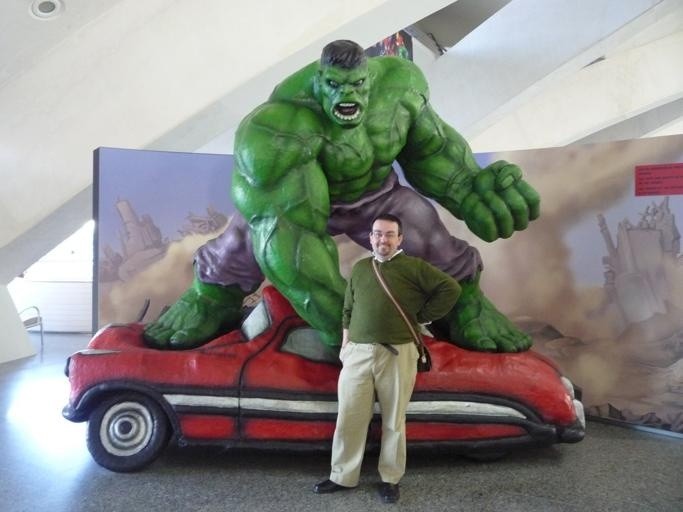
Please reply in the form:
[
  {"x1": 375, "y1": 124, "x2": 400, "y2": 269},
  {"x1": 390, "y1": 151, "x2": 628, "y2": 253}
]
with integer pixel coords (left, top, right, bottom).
[{"x1": 417, "y1": 343, "x2": 432, "y2": 372}]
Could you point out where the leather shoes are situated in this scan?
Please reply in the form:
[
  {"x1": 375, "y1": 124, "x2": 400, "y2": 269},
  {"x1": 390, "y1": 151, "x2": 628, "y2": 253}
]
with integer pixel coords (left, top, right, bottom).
[
  {"x1": 381, "y1": 482, "x2": 400, "y2": 504},
  {"x1": 312, "y1": 479, "x2": 358, "y2": 495}
]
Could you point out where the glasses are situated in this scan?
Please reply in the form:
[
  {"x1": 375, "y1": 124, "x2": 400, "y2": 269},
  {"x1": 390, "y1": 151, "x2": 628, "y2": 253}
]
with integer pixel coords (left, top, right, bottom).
[{"x1": 375, "y1": 231, "x2": 396, "y2": 241}]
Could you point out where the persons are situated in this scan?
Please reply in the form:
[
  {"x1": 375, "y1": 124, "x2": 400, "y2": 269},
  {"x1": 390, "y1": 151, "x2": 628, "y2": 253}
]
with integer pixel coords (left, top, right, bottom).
[
  {"x1": 140, "y1": 38, "x2": 540, "y2": 354},
  {"x1": 312, "y1": 214, "x2": 461, "y2": 503}
]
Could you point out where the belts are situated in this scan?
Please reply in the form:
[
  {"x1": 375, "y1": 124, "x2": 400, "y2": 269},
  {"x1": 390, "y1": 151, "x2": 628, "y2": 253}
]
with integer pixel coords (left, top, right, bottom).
[{"x1": 380, "y1": 343, "x2": 399, "y2": 355}]
